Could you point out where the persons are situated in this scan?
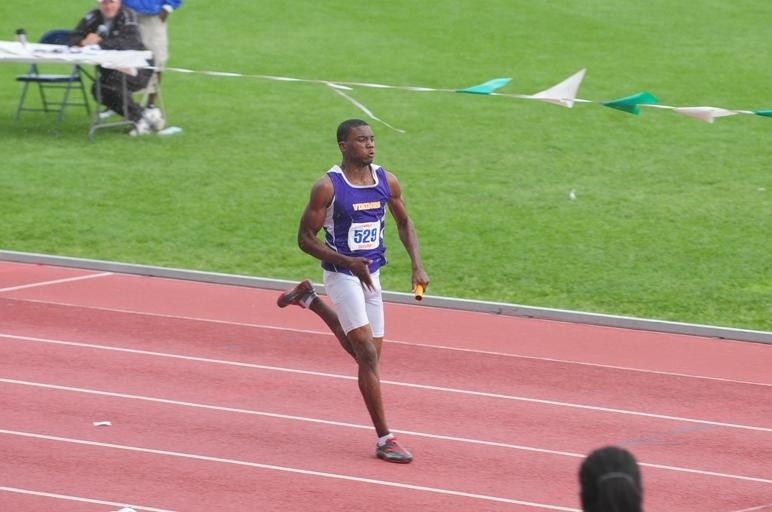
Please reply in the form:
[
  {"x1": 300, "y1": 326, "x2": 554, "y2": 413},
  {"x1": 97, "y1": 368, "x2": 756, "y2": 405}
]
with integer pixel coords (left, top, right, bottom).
[
  {"x1": 65, "y1": 0, "x2": 168, "y2": 135},
  {"x1": 577, "y1": 445, "x2": 644, "y2": 512},
  {"x1": 122, "y1": 0, "x2": 183, "y2": 135},
  {"x1": 279, "y1": 120, "x2": 431, "y2": 465}
]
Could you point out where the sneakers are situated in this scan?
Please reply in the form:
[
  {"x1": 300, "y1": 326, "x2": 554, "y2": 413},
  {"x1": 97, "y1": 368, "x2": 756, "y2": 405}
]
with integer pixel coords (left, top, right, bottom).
[
  {"x1": 129, "y1": 104, "x2": 165, "y2": 138},
  {"x1": 376, "y1": 438, "x2": 413, "y2": 463},
  {"x1": 277, "y1": 281, "x2": 315, "y2": 309}
]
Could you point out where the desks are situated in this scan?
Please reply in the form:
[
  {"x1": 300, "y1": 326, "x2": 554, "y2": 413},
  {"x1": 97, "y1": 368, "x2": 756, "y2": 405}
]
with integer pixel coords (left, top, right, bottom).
[{"x1": 0, "y1": 39, "x2": 152, "y2": 138}]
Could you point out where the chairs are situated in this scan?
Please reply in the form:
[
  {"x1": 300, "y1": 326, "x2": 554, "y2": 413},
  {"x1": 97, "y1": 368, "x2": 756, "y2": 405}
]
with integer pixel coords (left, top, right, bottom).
[
  {"x1": 12, "y1": 29, "x2": 91, "y2": 134},
  {"x1": 130, "y1": 32, "x2": 166, "y2": 128}
]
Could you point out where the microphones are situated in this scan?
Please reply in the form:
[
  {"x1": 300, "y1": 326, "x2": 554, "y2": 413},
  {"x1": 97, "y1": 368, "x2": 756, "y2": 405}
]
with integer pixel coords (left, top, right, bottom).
[{"x1": 95, "y1": 25, "x2": 107, "y2": 36}]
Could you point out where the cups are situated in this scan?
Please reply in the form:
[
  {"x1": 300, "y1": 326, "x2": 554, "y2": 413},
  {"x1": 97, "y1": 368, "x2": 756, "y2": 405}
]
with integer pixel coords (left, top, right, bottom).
[{"x1": 16, "y1": 28, "x2": 27, "y2": 51}]
